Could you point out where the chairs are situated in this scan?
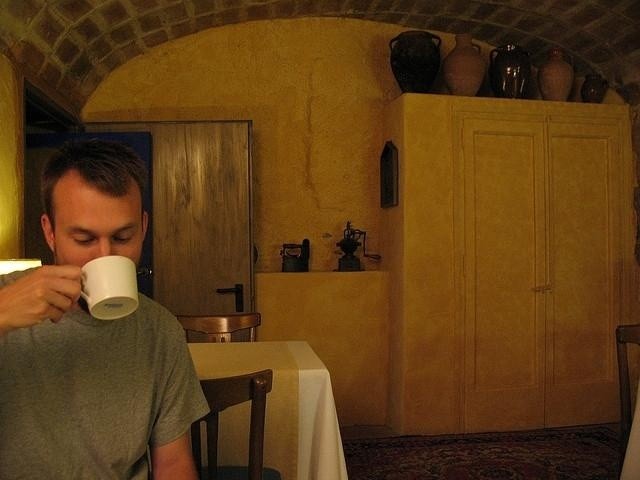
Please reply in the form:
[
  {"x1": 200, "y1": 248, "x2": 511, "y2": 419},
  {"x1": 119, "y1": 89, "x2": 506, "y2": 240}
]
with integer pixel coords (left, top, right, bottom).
[
  {"x1": 191, "y1": 369, "x2": 281, "y2": 480},
  {"x1": 616, "y1": 324, "x2": 640, "y2": 479},
  {"x1": 172, "y1": 313, "x2": 261, "y2": 342}
]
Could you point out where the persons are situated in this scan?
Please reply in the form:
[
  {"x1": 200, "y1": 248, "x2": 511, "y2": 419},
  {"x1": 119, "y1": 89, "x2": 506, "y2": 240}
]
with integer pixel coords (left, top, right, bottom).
[{"x1": 0, "y1": 137, "x2": 211, "y2": 480}]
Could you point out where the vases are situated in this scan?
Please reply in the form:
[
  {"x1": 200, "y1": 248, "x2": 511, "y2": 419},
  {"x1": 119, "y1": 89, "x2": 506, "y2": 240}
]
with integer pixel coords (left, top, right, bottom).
[
  {"x1": 537, "y1": 48, "x2": 573, "y2": 101},
  {"x1": 441, "y1": 33, "x2": 487, "y2": 97},
  {"x1": 581, "y1": 73, "x2": 608, "y2": 102},
  {"x1": 489, "y1": 42, "x2": 530, "y2": 99},
  {"x1": 389, "y1": 30, "x2": 441, "y2": 93}
]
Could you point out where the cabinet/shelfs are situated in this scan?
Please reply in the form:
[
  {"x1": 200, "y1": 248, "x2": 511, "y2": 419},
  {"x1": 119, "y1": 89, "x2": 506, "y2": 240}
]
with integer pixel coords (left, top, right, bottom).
[{"x1": 383, "y1": 92, "x2": 638, "y2": 434}]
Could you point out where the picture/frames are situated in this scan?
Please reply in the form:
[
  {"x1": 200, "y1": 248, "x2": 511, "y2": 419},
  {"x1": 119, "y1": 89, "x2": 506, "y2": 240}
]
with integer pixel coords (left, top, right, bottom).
[{"x1": 379, "y1": 141, "x2": 398, "y2": 208}]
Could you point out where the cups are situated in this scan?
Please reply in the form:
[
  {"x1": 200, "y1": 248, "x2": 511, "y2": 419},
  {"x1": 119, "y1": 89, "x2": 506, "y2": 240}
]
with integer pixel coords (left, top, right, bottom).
[{"x1": 80, "y1": 256, "x2": 140, "y2": 321}]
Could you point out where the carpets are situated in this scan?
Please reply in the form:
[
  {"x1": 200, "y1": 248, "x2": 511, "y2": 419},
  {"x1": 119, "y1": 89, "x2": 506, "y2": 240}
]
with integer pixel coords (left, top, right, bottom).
[{"x1": 342, "y1": 427, "x2": 622, "y2": 480}]
